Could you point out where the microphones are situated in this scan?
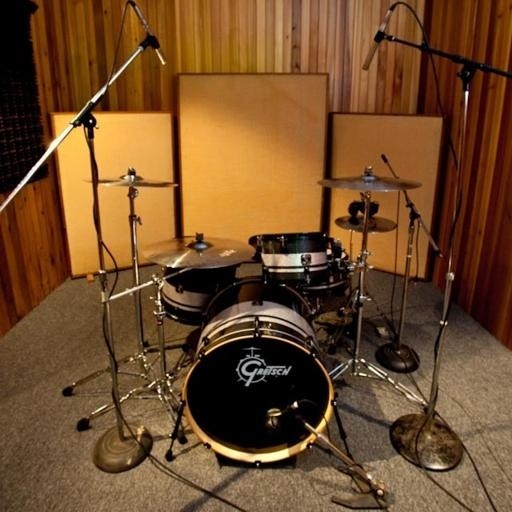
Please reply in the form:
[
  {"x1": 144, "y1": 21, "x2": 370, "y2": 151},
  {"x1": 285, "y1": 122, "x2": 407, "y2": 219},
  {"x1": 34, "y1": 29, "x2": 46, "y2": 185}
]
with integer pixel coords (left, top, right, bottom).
[
  {"x1": 362, "y1": 4, "x2": 396, "y2": 70},
  {"x1": 128, "y1": 0, "x2": 168, "y2": 66},
  {"x1": 265, "y1": 401, "x2": 299, "y2": 428}
]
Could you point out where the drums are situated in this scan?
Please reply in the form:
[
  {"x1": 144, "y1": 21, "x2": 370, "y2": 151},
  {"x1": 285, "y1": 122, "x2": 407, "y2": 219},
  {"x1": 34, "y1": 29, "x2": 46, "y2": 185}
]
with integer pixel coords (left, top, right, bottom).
[
  {"x1": 303, "y1": 251, "x2": 350, "y2": 293},
  {"x1": 156, "y1": 265, "x2": 238, "y2": 326},
  {"x1": 181, "y1": 276, "x2": 337, "y2": 468},
  {"x1": 254, "y1": 232, "x2": 331, "y2": 286}
]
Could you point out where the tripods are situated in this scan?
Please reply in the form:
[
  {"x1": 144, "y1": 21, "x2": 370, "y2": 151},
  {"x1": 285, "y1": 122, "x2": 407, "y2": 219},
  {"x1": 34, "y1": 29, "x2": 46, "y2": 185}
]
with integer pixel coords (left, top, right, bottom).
[
  {"x1": 62, "y1": 187, "x2": 188, "y2": 444},
  {"x1": 327, "y1": 191, "x2": 438, "y2": 418}
]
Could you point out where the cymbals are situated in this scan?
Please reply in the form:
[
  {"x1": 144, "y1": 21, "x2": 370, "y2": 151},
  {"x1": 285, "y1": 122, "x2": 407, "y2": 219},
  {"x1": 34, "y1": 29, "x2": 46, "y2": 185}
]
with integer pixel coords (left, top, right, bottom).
[
  {"x1": 335, "y1": 216, "x2": 398, "y2": 233},
  {"x1": 88, "y1": 180, "x2": 176, "y2": 186},
  {"x1": 140, "y1": 237, "x2": 256, "y2": 269},
  {"x1": 317, "y1": 177, "x2": 424, "y2": 192}
]
